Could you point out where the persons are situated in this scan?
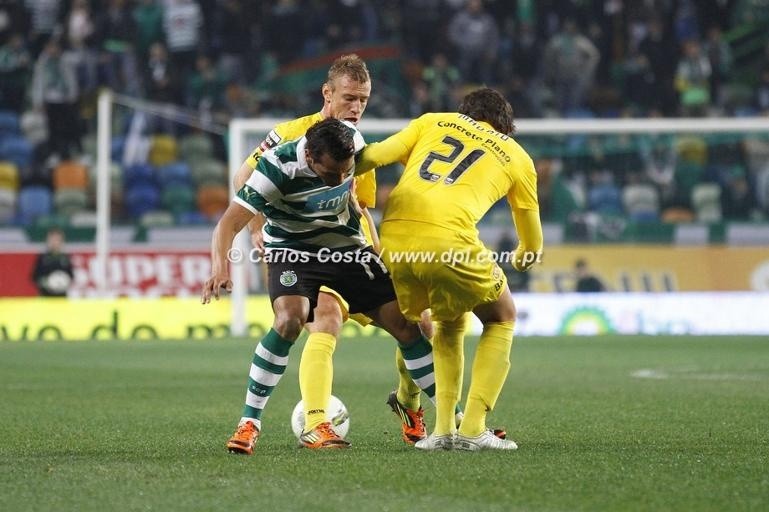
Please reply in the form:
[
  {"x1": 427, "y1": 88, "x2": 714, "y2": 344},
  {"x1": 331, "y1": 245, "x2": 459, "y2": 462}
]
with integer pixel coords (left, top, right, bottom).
[
  {"x1": 355, "y1": 88, "x2": 543, "y2": 451},
  {"x1": 32, "y1": 231, "x2": 73, "y2": 297},
  {"x1": 0, "y1": 1, "x2": 768, "y2": 221},
  {"x1": 201, "y1": 116, "x2": 505, "y2": 452},
  {"x1": 233, "y1": 54, "x2": 428, "y2": 448}
]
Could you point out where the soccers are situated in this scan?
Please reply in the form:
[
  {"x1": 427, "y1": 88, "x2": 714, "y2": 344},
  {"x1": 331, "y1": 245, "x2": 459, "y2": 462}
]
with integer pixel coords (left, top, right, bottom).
[{"x1": 291, "y1": 395, "x2": 349, "y2": 443}]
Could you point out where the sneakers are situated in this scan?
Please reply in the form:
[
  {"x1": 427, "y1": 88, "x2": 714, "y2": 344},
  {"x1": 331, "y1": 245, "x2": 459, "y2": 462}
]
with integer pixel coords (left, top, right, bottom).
[
  {"x1": 386, "y1": 390, "x2": 518, "y2": 450},
  {"x1": 227, "y1": 421, "x2": 259, "y2": 454},
  {"x1": 298, "y1": 421, "x2": 351, "y2": 448}
]
